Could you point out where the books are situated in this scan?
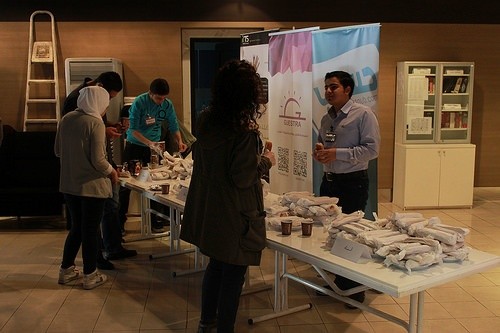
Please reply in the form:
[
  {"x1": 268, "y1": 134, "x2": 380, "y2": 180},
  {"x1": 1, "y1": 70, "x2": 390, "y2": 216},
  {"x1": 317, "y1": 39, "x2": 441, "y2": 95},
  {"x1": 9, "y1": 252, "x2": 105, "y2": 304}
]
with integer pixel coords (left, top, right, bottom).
[{"x1": 424, "y1": 76, "x2": 469, "y2": 129}]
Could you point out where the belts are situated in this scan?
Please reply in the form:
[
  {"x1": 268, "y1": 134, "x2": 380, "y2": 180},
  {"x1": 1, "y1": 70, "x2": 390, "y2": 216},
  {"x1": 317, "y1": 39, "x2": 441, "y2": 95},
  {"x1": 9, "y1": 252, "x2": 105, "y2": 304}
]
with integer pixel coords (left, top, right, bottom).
[{"x1": 324, "y1": 170, "x2": 367, "y2": 182}]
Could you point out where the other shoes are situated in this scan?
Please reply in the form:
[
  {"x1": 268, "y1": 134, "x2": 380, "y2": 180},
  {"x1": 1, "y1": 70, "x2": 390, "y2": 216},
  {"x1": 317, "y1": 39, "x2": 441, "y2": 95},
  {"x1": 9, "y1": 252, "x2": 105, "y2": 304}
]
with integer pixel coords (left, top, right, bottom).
[
  {"x1": 198, "y1": 318, "x2": 219, "y2": 332},
  {"x1": 315, "y1": 283, "x2": 349, "y2": 296},
  {"x1": 344, "y1": 295, "x2": 366, "y2": 310},
  {"x1": 105, "y1": 246, "x2": 137, "y2": 260},
  {"x1": 150, "y1": 222, "x2": 165, "y2": 233},
  {"x1": 95, "y1": 252, "x2": 114, "y2": 270}
]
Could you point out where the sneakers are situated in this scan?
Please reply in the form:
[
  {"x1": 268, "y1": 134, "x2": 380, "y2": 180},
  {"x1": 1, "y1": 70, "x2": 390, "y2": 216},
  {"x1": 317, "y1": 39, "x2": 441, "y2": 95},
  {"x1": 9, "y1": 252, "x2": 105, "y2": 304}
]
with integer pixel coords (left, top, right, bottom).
[
  {"x1": 82, "y1": 266, "x2": 108, "y2": 290},
  {"x1": 57, "y1": 264, "x2": 83, "y2": 285}
]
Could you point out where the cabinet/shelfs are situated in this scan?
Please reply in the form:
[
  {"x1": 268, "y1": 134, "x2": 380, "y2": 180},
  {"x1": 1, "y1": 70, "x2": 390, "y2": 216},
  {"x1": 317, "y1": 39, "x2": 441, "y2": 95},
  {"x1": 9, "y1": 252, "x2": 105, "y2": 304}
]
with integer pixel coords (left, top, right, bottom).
[{"x1": 392, "y1": 61, "x2": 476, "y2": 210}]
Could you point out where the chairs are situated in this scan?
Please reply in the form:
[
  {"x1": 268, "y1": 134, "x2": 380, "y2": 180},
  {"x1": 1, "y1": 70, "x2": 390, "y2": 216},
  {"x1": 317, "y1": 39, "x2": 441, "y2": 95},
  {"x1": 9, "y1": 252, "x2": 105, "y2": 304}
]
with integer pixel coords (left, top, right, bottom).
[{"x1": 0, "y1": 125, "x2": 62, "y2": 216}]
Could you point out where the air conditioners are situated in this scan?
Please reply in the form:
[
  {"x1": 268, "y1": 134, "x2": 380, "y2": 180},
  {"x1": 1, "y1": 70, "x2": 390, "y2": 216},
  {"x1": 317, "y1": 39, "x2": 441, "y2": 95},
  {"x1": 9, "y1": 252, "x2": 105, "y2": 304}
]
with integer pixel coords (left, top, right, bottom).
[{"x1": 65, "y1": 57, "x2": 124, "y2": 159}]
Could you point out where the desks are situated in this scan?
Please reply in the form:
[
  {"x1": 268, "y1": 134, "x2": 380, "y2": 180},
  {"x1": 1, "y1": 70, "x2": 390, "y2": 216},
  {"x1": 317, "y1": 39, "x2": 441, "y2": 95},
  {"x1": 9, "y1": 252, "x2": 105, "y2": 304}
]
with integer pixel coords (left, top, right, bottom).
[{"x1": 118, "y1": 166, "x2": 500, "y2": 333}]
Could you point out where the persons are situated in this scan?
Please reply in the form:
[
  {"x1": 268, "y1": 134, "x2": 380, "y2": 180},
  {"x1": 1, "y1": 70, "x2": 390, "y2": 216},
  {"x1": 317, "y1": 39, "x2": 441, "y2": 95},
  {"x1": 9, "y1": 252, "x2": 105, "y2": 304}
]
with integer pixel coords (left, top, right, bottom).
[
  {"x1": 315, "y1": 71, "x2": 382, "y2": 309},
  {"x1": 54, "y1": 71, "x2": 187, "y2": 290},
  {"x1": 188, "y1": 59, "x2": 275, "y2": 333}
]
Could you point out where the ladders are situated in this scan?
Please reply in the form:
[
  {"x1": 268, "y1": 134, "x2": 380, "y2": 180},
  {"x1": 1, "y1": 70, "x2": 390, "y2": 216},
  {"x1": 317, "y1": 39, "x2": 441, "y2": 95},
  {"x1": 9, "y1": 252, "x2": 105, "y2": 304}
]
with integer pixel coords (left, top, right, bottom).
[{"x1": 23, "y1": 11, "x2": 60, "y2": 131}]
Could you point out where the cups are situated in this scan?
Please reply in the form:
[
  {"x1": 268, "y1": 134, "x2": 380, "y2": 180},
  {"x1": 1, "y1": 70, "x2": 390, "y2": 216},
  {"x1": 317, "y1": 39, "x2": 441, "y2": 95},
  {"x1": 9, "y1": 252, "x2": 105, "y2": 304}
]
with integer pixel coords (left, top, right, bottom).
[
  {"x1": 280, "y1": 220, "x2": 292, "y2": 235},
  {"x1": 301, "y1": 220, "x2": 314, "y2": 236},
  {"x1": 161, "y1": 184, "x2": 169, "y2": 194}
]
